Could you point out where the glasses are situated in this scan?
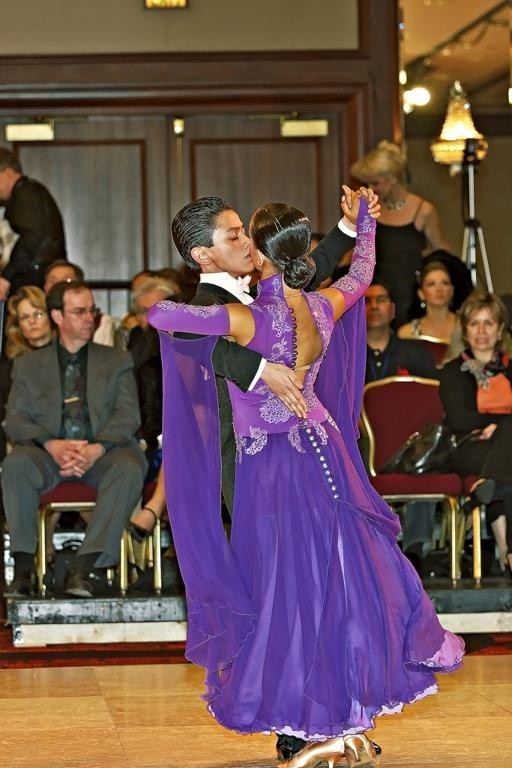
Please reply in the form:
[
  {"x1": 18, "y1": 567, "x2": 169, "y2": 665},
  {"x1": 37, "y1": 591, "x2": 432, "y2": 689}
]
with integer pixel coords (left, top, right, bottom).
[
  {"x1": 68, "y1": 308, "x2": 97, "y2": 315},
  {"x1": 20, "y1": 310, "x2": 45, "y2": 320}
]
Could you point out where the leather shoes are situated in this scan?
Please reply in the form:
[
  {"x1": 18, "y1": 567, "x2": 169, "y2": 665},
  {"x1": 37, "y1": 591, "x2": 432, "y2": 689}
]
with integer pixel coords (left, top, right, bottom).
[
  {"x1": 127, "y1": 507, "x2": 156, "y2": 542},
  {"x1": 63, "y1": 568, "x2": 93, "y2": 597},
  {"x1": 464, "y1": 478, "x2": 495, "y2": 516},
  {"x1": 3, "y1": 570, "x2": 39, "y2": 599}
]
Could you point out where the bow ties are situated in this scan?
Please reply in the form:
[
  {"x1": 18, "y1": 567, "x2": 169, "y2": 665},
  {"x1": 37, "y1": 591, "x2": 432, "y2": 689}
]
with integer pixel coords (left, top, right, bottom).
[{"x1": 237, "y1": 274, "x2": 252, "y2": 295}]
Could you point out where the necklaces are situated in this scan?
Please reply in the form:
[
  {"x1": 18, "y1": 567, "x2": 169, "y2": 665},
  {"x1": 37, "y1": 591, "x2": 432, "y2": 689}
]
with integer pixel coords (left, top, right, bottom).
[{"x1": 382, "y1": 186, "x2": 408, "y2": 210}]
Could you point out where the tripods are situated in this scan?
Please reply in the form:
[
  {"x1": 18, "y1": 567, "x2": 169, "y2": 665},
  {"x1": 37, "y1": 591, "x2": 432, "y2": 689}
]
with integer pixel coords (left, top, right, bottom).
[{"x1": 457, "y1": 146, "x2": 497, "y2": 297}]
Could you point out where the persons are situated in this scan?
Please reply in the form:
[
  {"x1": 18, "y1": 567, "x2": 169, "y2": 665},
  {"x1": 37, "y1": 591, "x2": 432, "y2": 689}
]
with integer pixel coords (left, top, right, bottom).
[
  {"x1": 146, "y1": 192, "x2": 467, "y2": 768},
  {"x1": 0, "y1": 147, "x2": 68, "y2": 306},
  {"x1": 174, "y1": 185, "x2": 384, "y2": 756},
  {"x1": 349, "y1": 141, "x2": 447, "y2": 329},
  {"x1": 0, "y1": 234, "x2": 512, "y2": 598}
]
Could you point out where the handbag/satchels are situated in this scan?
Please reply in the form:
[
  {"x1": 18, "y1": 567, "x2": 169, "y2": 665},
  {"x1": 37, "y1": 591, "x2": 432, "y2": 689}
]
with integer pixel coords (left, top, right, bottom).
[{"x1": 402, "y1": 423, "x2": 456, "y2": 476}]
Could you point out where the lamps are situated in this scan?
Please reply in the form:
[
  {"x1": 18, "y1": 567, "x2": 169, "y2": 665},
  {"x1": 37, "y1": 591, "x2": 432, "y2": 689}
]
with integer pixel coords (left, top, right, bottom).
[{"x1": 431, "y1": 40, "x2": 489, "y2": 177}]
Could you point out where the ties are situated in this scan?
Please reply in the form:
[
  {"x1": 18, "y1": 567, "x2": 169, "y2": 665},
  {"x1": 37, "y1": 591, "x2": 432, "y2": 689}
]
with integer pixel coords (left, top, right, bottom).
[{"x1": 64, "y1": 355, "x2": 87, "y2": 439}]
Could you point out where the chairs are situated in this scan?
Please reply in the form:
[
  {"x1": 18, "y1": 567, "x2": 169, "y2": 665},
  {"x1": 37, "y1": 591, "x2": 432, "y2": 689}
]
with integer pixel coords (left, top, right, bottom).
[
  {"x1": 361, "y1": 334, "x2": 483, "y2": 584},
  {"x1": 39, "y1": 480, "x2": 170, "y2": 595}
]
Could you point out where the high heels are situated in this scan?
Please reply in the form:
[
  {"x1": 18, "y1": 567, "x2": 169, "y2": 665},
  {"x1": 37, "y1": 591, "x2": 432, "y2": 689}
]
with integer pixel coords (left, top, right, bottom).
[
  {"x1": 344, "y1": 734, "x2": 380, "y2": 768},
  {"x1": 277, "y1": 736, "x2": 345, "y2": 767}
]
